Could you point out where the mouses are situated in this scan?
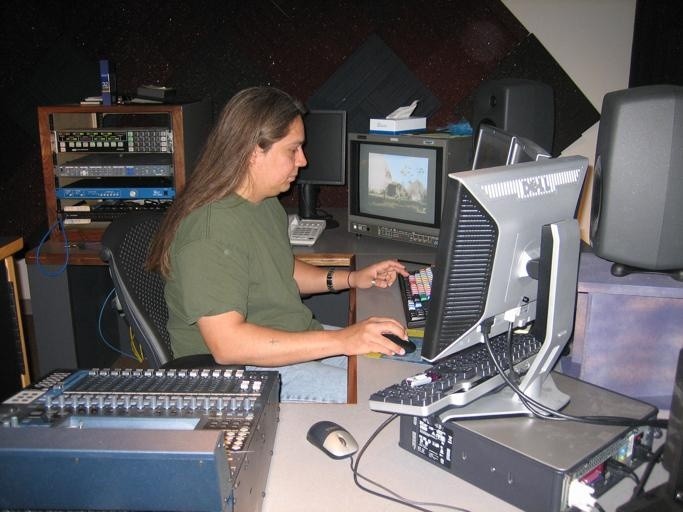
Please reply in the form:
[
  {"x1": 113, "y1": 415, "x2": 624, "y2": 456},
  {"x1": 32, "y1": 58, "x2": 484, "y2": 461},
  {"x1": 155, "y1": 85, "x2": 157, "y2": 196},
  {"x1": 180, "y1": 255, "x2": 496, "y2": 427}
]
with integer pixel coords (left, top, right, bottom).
[
  {"x1": 307, "y1": 420, "x2": 359, "y2": 460},
  {"x1": 382, "y1": 334, "x2": 416, "y2": 353}
]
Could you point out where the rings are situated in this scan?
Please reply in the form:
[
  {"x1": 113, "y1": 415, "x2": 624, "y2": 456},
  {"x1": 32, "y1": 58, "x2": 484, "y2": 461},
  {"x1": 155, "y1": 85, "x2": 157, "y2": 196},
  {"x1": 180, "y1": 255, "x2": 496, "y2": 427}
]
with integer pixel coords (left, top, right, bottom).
[{"x1": 372, "y1": 279, "x2": 376, "y2": 287}]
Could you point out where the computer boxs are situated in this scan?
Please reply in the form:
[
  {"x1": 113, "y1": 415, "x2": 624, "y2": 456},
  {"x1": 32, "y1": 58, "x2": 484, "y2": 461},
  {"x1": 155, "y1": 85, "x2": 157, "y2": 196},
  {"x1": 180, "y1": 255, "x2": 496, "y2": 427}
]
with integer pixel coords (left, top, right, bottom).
[{"x1": 401, "y1": 371, "x2": 658, "y2": 512}]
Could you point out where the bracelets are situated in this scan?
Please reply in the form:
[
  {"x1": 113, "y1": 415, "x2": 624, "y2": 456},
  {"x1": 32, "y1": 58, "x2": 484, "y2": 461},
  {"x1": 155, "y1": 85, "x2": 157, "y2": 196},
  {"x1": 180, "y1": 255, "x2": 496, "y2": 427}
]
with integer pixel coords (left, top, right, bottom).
[
  {"x1": 347, "y1": 270, "x2": 353, "y2": 288},
  {"x1": 327, "y1": 269, "x2": 336, "y2": 293}
]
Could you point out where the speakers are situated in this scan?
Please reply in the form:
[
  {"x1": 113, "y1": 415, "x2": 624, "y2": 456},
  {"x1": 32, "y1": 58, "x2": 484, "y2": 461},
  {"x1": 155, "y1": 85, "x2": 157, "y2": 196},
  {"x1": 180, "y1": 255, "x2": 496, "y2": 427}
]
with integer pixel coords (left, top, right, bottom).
[
  {"x1": 589, "y1": 84, "x2": 683, "y2": 282},
  {"x1": 473, "y1": 77, "x2": 554, "y2": 156}
]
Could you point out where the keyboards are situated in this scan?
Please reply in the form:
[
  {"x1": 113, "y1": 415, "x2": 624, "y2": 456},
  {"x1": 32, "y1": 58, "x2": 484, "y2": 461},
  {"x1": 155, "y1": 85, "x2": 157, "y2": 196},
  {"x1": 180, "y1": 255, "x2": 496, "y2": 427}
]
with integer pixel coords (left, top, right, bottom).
[
  {"x1": 289, "y1": 219, "x2": 327, "y2": 246},
  {"x1": 397, "y1": 263, "x2": 433, "y2": 329},
  {"x1": 369, "y1": 332, "x2": 543, "y2": 418}
]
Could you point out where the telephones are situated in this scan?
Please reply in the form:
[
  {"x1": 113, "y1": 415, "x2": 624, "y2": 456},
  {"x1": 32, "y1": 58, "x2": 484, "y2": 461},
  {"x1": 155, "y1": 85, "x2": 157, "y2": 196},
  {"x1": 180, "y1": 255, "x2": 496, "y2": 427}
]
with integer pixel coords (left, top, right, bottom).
[{"x1": 288, "y1": 214, "x2": 327, "y2": 246}]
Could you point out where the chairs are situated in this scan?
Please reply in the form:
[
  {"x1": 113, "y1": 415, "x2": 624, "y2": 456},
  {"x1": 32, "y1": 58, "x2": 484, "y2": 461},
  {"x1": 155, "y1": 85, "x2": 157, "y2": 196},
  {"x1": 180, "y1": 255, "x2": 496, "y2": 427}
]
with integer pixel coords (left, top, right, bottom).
[{"x1": 97, "y1": 208, "x2": 247, "y2": 370}]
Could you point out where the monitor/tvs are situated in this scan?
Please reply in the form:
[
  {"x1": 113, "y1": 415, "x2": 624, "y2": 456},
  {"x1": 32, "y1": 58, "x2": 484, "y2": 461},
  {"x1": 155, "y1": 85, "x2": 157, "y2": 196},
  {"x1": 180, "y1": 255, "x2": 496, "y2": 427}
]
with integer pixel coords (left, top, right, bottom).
[
  {"x1": 471, "y1": 123, "x2": 518, "y2": 172},
  {"x1": 347, "y1": 129, "x2": 473, "y2": 248},
  {"x1": 291, "y1": 110, "x2": 347, "y2": 229},
  {"x1": 509, "y1": 136, "x2": 551, "y2": 167},
  {"x1": 421, "y1": 155, "x2": 588, "y2": 424}
]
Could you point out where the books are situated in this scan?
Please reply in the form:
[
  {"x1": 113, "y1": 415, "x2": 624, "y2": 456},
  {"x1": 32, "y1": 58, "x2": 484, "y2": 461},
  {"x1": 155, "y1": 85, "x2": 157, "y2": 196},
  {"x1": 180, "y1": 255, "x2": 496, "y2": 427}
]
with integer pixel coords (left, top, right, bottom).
[{"x1": 81, "y1": 97, "x2": 103, "y2": 104}]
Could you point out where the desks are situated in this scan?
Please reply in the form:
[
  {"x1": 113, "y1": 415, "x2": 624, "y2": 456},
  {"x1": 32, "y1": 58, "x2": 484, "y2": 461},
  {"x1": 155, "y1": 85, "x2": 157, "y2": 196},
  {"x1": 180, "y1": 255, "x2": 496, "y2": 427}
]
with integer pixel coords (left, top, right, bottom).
[
  {"x1": 257, "y1": 206, "x2": 526, "y2": 508},
  {"x1": 26, "y1": 233, "x2": 133, "y2": 374}
]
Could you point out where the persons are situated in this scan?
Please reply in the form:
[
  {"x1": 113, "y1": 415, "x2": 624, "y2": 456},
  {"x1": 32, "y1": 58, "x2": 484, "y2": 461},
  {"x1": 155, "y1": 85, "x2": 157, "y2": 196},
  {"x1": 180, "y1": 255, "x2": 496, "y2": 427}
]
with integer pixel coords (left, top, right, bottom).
[{"x1": 144, "y1": 87, "x2": 409, "y2": 367}]
